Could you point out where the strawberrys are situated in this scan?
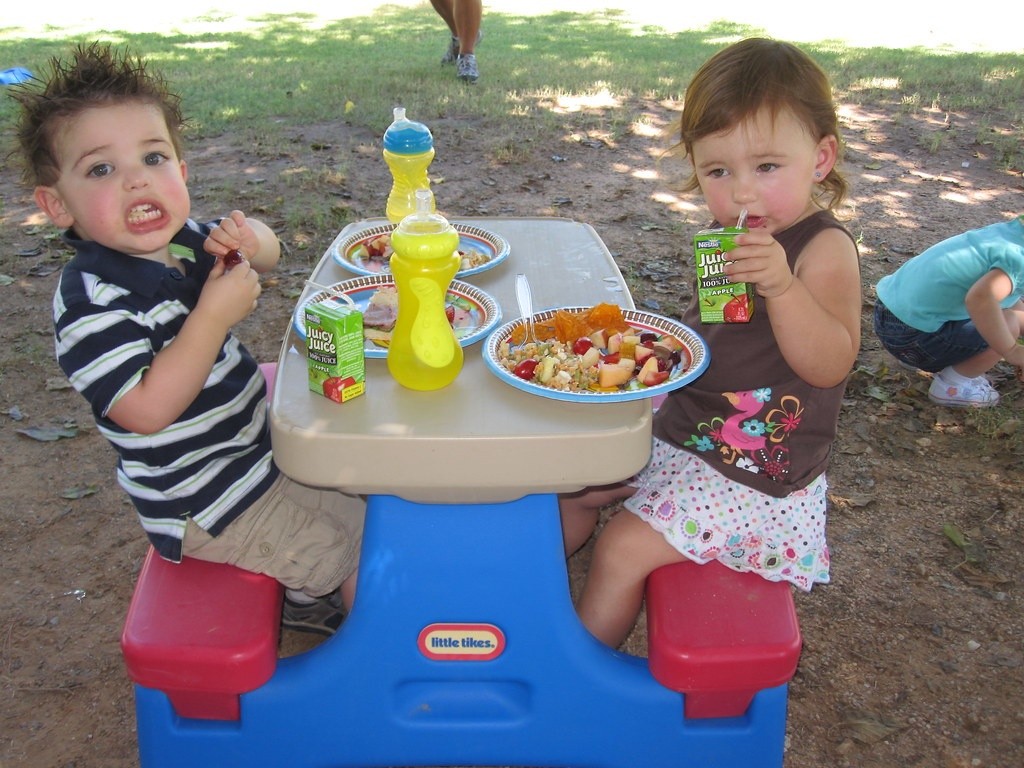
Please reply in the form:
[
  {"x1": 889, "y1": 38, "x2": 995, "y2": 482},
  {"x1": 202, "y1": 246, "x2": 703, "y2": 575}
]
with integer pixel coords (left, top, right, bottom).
[{"x1": 644, "y1": 371, "x2": 668, "y2": 386}]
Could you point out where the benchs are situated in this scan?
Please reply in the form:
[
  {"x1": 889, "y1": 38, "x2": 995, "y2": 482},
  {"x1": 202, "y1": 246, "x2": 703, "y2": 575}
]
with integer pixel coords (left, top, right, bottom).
[
  {"x1": 643, "y1": 558, "x2": 802, "y2": 718},
  {"x1": 121, "y1": 361, "x2": 283, "y2": 723}
]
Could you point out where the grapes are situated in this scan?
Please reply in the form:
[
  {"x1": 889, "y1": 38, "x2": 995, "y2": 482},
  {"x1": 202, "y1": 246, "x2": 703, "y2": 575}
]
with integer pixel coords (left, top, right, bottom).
[
  {"x1": 573, "y1": 336, "x2": 592, "y2": 355},
  {"x1": 513, "y1": 358, "x2": 538, "y2": 379},
  {"x1": 223, "y1": 249, "x2": 244, "y2": 269}
]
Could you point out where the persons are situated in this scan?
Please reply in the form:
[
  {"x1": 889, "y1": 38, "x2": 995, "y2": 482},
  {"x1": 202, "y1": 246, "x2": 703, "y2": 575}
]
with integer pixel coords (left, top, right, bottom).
[
  {"x1": 9, "y1": 38, "x2": 366, "y2": 613},
  {"x1": 558, "y1": 39, "x2": 861, "y2": 648},
  {"x1": 874, "y1": 214, "x2": 1024, "y2": 407},
  {"x1": 430, "y1": 0, "x2": 483, "y2": 82}
]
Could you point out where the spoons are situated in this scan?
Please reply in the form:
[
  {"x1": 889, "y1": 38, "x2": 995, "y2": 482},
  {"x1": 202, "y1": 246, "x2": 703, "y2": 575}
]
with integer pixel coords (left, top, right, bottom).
[{"x1": 514, "y1": 272, "x2": 541, "y2": 349}]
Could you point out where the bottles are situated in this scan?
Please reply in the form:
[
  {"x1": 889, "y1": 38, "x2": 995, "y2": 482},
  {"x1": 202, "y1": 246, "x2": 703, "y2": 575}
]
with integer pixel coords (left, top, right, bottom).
[
  {"x1": 388, "y1": 188, "x2": 464, "y2": 391},
  {"x1": 381, "y1": 106, "x2": 437, "y2": 223}
]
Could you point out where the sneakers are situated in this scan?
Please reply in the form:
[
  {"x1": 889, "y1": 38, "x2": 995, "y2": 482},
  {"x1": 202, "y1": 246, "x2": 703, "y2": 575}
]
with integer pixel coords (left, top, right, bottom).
[
  {"x1": 455, "y1": 53, "x2": 480, "y2": 81},
  {"x1": 281, "y1": 589, "x2": 345, "y2": 635},
  {"x1": 441, "y1": 30, "x2": 483, "y2": 67},
  {"x1": 928, "y1": 371, "x2": 1000, "y2": 408}
]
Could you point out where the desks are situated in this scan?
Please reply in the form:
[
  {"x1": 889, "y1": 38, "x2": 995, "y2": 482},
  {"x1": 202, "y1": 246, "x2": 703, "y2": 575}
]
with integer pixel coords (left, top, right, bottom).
[{"x1": 137, "y1": 219, "x2": 787, "y2": 766}]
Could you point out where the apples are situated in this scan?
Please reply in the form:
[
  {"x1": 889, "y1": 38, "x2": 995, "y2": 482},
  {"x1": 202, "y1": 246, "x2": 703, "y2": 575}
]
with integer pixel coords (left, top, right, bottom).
[
  {"x1": 309, "y1": 368, "x2": 356, "y2": 403},
  {"x1": 699, "y1": 292, "x2": 751, "y2": 322}
]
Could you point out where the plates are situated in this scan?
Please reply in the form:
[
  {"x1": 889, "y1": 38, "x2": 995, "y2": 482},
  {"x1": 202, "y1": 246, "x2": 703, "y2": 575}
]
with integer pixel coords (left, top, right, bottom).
[
  {"x1": 482, "y1": 305, "x2": 711, "y2": 406},
  {"x1": 331, "y1": 220, "x2": 511, "y2": 281},
  {"x1": 293, "y1": 273, "x2": 504, "y2": 360}
]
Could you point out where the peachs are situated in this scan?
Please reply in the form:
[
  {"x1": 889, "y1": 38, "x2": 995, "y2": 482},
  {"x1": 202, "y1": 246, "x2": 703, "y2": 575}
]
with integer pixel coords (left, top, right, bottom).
[{"x1": 582, "y1": 328, "x2": 681, "y2": 386}]
